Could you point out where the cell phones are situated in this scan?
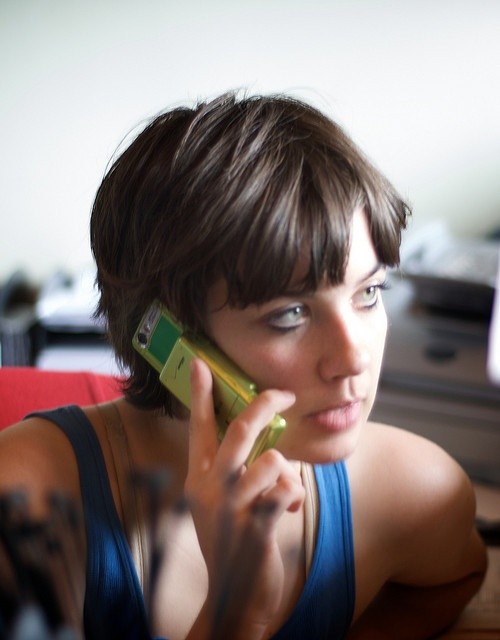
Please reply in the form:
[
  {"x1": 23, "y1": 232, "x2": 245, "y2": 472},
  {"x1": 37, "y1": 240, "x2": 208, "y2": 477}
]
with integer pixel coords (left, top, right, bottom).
[{"x1": 132, "y1": 297, "x2": 287, "y2": 468}]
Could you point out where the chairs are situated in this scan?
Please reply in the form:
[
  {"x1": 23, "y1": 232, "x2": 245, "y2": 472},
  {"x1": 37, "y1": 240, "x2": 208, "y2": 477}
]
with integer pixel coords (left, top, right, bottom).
[{"x1": 3, "y1": 367, "x2": 126, "y2": 429}]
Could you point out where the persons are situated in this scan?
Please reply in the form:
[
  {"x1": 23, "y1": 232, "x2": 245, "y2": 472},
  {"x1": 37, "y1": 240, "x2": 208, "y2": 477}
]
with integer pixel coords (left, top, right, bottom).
[{"x1": 1, "y1": 89, "x2": 489, "y2": 640}]
